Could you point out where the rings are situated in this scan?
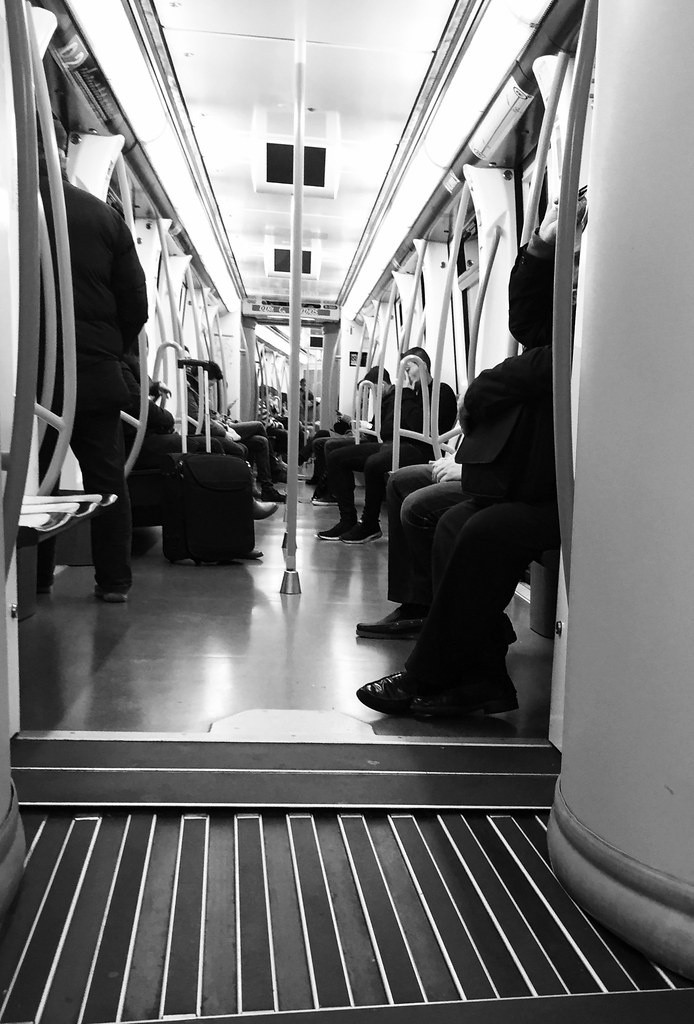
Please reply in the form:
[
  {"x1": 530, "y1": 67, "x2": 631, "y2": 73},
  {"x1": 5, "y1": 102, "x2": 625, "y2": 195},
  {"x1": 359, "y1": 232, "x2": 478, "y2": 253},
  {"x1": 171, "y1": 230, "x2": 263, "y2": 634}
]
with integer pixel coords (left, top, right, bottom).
[{"x1": 553, "y1": 198, "x2": 558, "y2": 204}]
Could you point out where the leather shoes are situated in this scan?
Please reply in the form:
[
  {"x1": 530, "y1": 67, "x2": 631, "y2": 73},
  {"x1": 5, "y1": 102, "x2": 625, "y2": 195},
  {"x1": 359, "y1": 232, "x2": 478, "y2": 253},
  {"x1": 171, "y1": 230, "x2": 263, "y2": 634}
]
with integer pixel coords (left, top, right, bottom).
[
  {"x1": 356, "y1": 670, "x2": 443, "y2": 714},
  {"x1": 409, "y1": 674, "x2": 519, "y2": 718}
]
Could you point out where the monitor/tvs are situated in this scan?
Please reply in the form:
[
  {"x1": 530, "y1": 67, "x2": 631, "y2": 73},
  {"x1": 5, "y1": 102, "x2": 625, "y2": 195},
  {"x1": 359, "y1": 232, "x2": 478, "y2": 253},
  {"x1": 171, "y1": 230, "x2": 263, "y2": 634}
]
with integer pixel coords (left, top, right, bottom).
[
  {"x1": 310, "y1": 337, "x2": 323, "y2": 347},
  {"x1": 265, "y1": 143, "x2": 326, "y2": 188},
  {"x1": 274, "y1": 249, "x2": 311, "y2": 274}
]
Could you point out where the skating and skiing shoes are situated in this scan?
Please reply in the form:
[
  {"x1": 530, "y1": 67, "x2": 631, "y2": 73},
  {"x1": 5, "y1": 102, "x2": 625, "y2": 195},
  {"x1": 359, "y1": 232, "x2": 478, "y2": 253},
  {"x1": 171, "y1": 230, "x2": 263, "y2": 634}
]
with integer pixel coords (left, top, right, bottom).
[{"x1": 318, "y1": 520, "x2": 357, "y2": 540}]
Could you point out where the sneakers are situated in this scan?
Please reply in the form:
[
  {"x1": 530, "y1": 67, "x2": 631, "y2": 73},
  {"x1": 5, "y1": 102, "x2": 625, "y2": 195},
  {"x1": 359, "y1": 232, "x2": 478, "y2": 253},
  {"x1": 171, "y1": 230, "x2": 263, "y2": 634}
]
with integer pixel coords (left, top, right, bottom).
[{"x1": 341, "y1": 520, "x2": 382, "y2": 544}]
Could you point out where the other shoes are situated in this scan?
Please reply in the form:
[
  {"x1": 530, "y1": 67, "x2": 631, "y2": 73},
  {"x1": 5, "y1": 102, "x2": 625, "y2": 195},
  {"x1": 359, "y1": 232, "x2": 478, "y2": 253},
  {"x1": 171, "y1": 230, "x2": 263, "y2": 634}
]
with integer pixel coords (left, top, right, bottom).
[
  {"x1": 261, "y1": 489, "x2": 286, "y2": 502},
  {"x1": 36, "y1": 585, "x2": 54, "y2": 594},
  {"x1": 306, "y1": 479, "x2": 317, "y2": 485},
  {"x1": 245, "y1": 549, "x2": 263, "y2": 559},
  {"x1": 94, "y1": 584, "x2": 128, "y2": 601},
  {"x1": 165, "y1": 557, "x2": 198, "y2": 566},
  {"x1": 252, "y1": 480, "x2": 262, "y2": 498},
  {"x1": 252, "y1": 497, "x2": 278, "y2": 519},
  {"x1": 312, "y1": 494, "x2": 338, "y2": 506},
  {"x1": 357, "y1": 606, "x2": 427, "y2": 638}
]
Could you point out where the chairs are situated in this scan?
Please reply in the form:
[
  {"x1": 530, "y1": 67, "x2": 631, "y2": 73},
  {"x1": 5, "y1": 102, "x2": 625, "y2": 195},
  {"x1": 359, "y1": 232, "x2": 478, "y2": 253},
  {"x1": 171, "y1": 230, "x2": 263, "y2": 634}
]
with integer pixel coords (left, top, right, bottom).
[{"x1": 17, "y1": 495, "x2": 119, "y2": 542}]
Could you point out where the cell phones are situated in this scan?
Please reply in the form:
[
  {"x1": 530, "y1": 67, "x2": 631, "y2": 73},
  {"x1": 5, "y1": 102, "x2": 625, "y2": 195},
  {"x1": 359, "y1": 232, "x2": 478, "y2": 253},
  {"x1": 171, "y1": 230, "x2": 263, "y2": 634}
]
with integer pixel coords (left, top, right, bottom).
[{"x1": 335, "y1": 410, "x2": 343, "y2": 416}]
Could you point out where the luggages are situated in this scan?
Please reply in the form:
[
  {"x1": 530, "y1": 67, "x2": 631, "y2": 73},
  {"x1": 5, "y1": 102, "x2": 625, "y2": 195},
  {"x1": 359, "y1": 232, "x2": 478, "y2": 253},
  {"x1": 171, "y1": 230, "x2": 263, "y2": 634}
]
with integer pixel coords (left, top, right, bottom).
[{"x1": 161, "y1": 359, "x2": 256, "y2": 565}]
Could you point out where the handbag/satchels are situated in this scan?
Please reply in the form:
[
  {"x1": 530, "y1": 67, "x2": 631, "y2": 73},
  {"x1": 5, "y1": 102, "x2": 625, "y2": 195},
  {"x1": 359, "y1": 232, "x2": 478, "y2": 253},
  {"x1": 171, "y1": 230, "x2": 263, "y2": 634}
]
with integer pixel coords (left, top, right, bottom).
[{"x1": 454, "y1": 398, "x2": 537, "y2": 498}]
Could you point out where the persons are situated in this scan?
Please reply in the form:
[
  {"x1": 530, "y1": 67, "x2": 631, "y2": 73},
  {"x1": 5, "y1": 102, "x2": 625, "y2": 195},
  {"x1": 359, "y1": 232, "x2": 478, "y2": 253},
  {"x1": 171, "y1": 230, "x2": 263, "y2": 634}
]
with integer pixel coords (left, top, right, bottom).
[
  {"x1": 356, "y1": 197, "x2": 587, "y2": 717},
  {"x1": 355, "y1": 450, "x2": 463, "y2": 638},
  {"x1": 186, "y1": 361, "x2": 287, "y2": 503},
  {"x1": 299, "y1": 366, "x2": 395, "y2": 505},
  {"x1": 300, "y1": 378, "x2": 314, "y2": 423},
  {"x1": 318, "y1": 347, "x2": 457, "y2": 542},
  {"x1": 121, "y1": 330, "x2": 278, "y2": 564},
  {"x1": 34, "y1": 108, "x2": 149, "y2": 602},
  {"x1": 257, "y1": 385, "x2": 288, "y2": 464}
]
